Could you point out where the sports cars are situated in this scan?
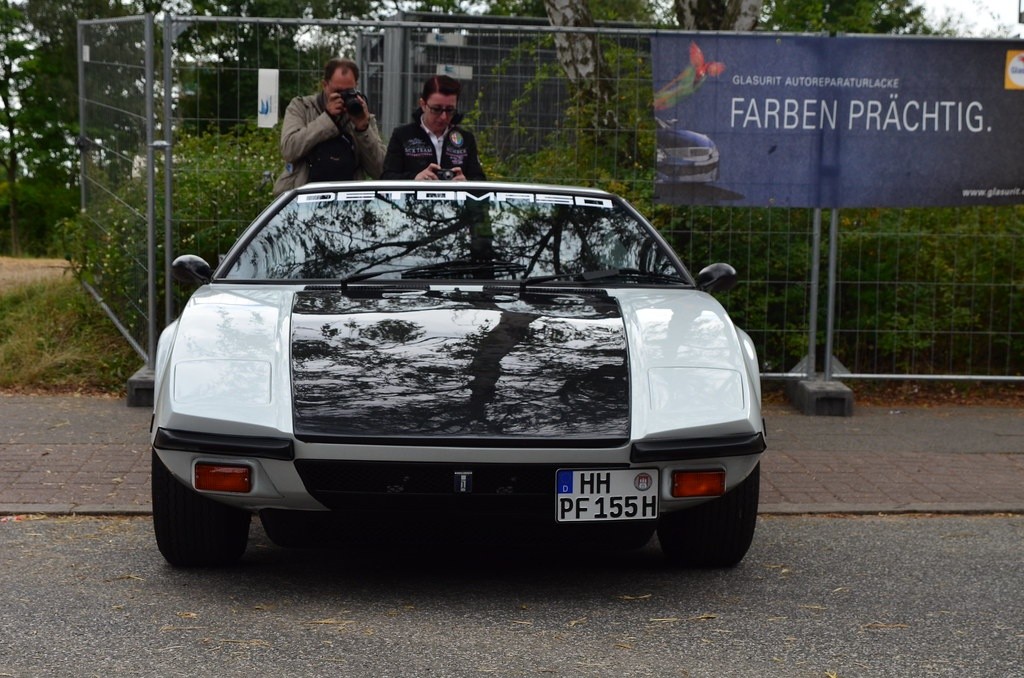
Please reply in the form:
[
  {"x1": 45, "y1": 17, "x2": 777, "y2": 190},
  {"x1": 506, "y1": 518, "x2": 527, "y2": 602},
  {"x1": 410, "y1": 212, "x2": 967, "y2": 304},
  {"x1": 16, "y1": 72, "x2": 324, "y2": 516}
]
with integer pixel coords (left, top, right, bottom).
[{"x1": 151, "y1": 180, "x2": 769, "y2": 572}]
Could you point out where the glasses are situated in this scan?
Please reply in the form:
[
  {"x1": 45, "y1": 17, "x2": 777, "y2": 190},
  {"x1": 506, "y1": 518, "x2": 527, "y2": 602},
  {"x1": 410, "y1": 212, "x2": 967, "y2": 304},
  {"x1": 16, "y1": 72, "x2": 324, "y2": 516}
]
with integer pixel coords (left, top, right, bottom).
[{"x1": 425, "y1": 104, "x2": 457, "y2": 114}]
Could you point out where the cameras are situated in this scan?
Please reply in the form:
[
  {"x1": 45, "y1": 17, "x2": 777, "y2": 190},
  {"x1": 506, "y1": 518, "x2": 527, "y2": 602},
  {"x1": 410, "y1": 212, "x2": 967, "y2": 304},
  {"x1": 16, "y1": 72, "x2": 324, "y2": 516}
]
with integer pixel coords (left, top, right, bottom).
[
  {"x1": 433, "y1": 168, "x2": 456, "y2": 181},
  {"x1": 337, "y1": 89, "x2": 363, "y2": 116}
]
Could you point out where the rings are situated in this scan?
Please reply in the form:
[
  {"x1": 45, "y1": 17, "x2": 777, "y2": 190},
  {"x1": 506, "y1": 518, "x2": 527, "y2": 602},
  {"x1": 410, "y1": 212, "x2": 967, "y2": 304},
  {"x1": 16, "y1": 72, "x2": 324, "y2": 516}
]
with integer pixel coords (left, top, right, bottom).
[{"x1": 424, "y1": 174, "x2": 431, "y2": 179}]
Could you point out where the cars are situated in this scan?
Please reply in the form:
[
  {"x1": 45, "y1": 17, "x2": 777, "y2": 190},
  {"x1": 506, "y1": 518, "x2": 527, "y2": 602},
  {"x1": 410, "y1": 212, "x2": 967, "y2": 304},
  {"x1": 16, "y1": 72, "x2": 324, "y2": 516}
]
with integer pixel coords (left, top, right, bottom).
[{"x1": 653, "y1": 116, "x2": 719, "y2": 191}]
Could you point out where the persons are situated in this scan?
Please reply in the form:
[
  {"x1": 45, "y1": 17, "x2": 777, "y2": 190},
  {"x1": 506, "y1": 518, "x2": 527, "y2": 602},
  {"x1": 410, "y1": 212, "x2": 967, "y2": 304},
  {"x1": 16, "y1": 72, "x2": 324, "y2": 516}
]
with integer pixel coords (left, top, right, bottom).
[
  {"x1": 280, "y1": 59, "x2": 386, "y2": 189},
  {"x1": 382, "y1": 75, "x2": 488, "y2": 182}
]
